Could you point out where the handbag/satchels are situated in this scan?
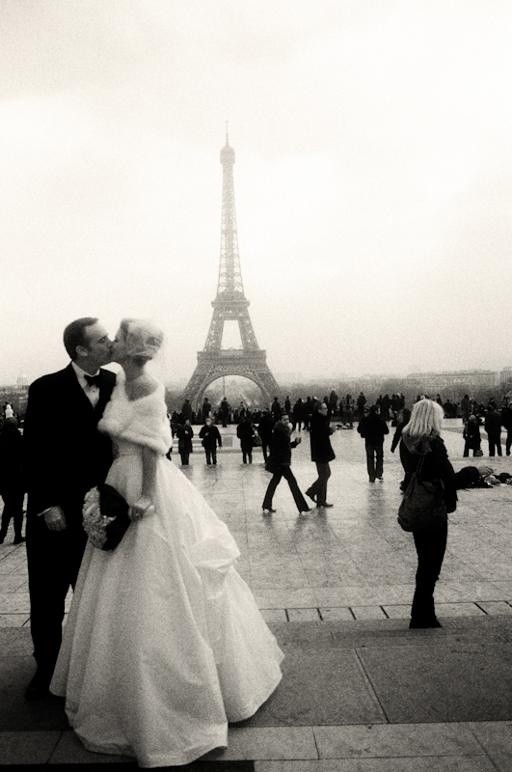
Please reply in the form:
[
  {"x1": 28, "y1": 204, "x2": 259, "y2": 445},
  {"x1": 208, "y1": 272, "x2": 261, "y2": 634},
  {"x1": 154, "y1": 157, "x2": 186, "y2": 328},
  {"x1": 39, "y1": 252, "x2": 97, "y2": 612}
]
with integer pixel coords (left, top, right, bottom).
[
  {"x1": 397, "y1": 454, "x2": 438, "y2": 531},
  {"x1": 263, "y1": 456, "x2": 289, "y2": 475}
]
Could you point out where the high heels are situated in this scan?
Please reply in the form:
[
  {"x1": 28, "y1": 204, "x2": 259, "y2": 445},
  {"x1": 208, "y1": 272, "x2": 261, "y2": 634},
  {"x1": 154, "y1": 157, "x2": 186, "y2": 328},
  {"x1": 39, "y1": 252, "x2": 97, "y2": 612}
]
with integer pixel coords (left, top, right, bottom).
[
  {"x1": 300, "y1": 509, "x2": 311, "y2": 513},
  {"x1": 263, "y1": 507, "x2": 276, "y2": 512}
]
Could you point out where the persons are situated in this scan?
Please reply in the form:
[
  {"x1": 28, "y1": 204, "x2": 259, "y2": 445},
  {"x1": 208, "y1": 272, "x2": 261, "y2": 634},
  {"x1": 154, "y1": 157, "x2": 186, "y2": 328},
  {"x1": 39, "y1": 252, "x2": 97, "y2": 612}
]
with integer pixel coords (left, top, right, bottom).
[
  {"x1": 49, "y1": 318, "x2": 285, "y2": 768},
  {"x1": 167, "y1": 390, "x2": 512, "y2": 515},
  {"x1": 23, "y1": 317, "x2": 112, "y2": 708},
  {"x1": 0, "y1": 403, "x2": 27, "y2": 545},
  {"x1": 398, "y1": 399, "x2": 493, "y2": 629}
]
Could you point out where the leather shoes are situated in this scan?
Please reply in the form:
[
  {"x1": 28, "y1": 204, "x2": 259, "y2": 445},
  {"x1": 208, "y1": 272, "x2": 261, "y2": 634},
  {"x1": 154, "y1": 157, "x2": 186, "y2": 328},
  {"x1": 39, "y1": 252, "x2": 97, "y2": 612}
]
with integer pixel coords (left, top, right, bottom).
[
  {"x1": 317, "y1": 502, "x2": 333, "y2": 507},
  {"x1": 305, "y1": 490, "x2": 317, "y2": 503}
]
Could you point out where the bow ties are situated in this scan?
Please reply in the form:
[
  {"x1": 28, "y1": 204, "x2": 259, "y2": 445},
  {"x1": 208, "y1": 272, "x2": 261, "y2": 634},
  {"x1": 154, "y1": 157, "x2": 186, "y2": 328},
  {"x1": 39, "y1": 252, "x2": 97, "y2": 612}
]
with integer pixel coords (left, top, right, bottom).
[{"x1": 84, "y1": 374, "x2": 101, "y2": 387}]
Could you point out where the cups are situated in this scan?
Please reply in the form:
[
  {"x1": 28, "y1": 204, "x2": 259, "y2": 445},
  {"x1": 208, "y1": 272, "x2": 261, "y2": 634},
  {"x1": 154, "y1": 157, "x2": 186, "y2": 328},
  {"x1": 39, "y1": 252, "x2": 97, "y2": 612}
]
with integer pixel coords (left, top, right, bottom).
[{"x1": 296, "y1": 436, "x2": 301, "y2": 444}]
